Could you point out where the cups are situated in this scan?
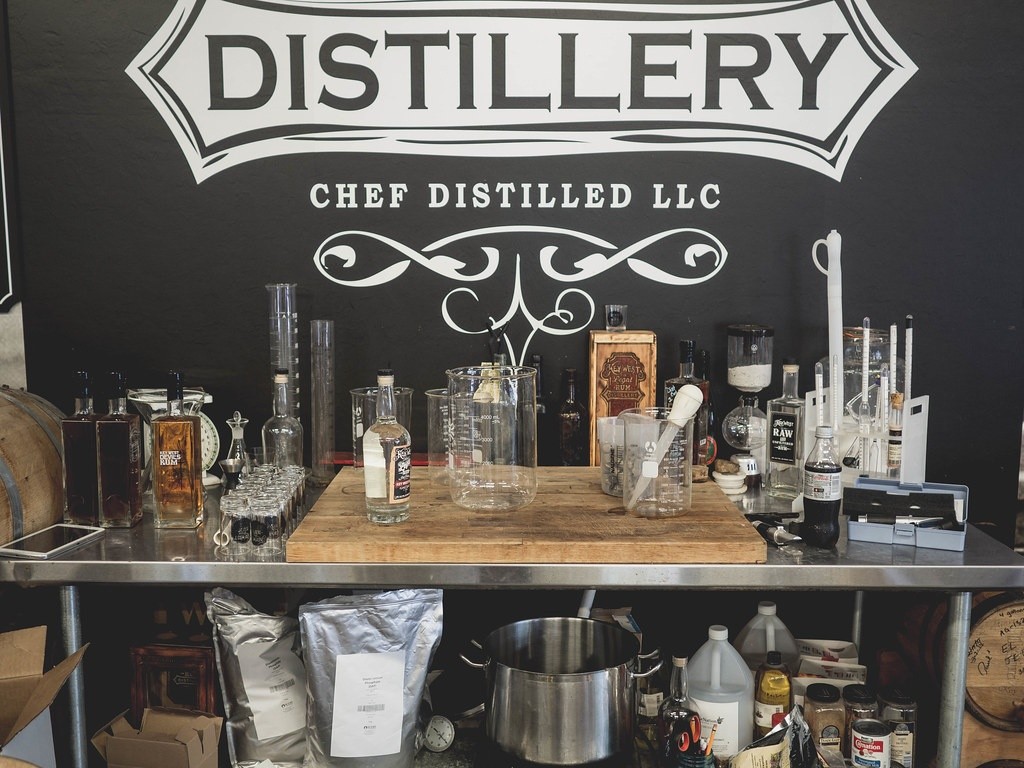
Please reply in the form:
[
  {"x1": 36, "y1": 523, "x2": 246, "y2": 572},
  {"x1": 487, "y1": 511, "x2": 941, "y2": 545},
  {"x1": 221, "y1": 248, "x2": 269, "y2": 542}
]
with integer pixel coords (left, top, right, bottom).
[
  {"x1": 425, "y1": 388, "x2": 454, "y2": 466},
  {"x1": 596, "y1": 416, "x2": 660, "y2": 498},
  {"x1": 605, "y1": 305, "x2": 628, "y2": 332},
  {"x1": 219, "y1": 464, "x2": 307, "y2": 557},
  {"x1": 446, "y1": 361, "x2": 538, "y2": 510},
  {"x1": 617, "y1": 406, "x2": 698, "y2": 518},
  {"x1": 349, "y1": 387, "x2": 414, "y2": 468}
]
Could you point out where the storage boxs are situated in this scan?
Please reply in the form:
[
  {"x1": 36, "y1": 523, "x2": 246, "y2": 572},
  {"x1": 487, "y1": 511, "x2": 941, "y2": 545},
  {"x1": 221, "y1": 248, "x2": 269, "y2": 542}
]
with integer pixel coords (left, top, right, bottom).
[
  {"x1": 590, "y1": 607, "x2": 642, "y2": 693},
  {"x1": 0, "y1": 622, "x2": 224, "y2": 768},
  {"x1": 790, "y1": 639, "x2": 867, "y2": 708},
  {"x1": 848, "y1": 476, "x2": 970, "y2": 551}
]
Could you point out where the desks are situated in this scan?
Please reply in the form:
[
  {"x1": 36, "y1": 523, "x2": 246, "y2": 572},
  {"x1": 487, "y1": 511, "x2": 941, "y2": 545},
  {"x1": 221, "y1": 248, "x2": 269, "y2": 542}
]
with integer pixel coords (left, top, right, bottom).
[{"x1": 0, "y1": 485, "x2": 1024, "y2": 768}]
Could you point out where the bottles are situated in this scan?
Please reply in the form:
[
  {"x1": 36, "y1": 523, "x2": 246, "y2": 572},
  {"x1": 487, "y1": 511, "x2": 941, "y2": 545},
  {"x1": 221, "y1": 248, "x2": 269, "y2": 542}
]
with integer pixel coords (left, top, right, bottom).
[
  {"x1": 658, "y1": 652, "x2": 701, "y2": 768},
  {"x1": 60, "y1": 371, "x2": 105, "y2": 526},
  {"x1": 635, "y1": 640, "x2": 670, "y2": 751},
  {"x1": 804, "y1": 426, "x2": 842, "y2": 548},
  {"x1": 362, "y1": 369, "x2": 411, "y2": 523},
  {"x1": 687, "y1": 625, "x2": 755, "y2": 759},
  {"x1": 755, "y1": 650, "x2": 794, "y2": 741},
  {"x1": 261, "y1": 368, "x2": 303, "y2": 465},
  {"x1": 819, "y1": 326, "x2": 905, "y2": 437},
  {"x1": 531, "y1": 355, "x2": 545, "y2": 435},
  {"x1": 880, "y1": 696, "x2": 917, "y2": 767},
  {"x1": 843, "y1": 683, "x2": 879, "y2": 754},
  {"x1": 698, "y1": 350, "x2": 718, "y2": 480},
  {"x1": 559, "y1": 369, "x2": 588, "y2": 467},
  {"x1": 219, "y1": 458, "x2": 245, "y2": 497},
  {"x1": 766, "y1": 365, "x2": 806, "y2": 498},
  {"x1": 219, "y1": 410, "x2": 251, "y2": 484},
  {"x1": 680, "y1": 748, "x2": 717, "y2": 768},
  {"x1": 663, "y1": 340, "x2": 710, "y2": 464},
  {"x1": 96, "y1": 370, "x2": 143, "y2": 528},
  {"x1": 151, "y1": 370, "x2": 204, "y2": 528},
  {"x1": 804, "y1": 683, "x2": 846, "y2": 752},
  {"x1": 733, "y1": 601, "x2": 800, "y2": 677}
]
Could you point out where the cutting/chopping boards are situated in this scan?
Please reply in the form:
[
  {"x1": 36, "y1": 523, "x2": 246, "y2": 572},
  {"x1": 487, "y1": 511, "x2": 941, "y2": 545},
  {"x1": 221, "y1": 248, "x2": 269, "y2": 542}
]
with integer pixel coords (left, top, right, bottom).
[{"x1": 286, "y1": 464, "x2": 769, "y2": 564}]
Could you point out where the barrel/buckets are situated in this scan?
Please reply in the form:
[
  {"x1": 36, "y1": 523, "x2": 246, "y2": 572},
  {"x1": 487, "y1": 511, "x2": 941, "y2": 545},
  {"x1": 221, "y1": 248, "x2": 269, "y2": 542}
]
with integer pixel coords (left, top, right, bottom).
[
  {"x1": 0, "y1": 388, "x2": 68, "y2": 550},
  {"x1": 898, "y1": 589, "x2": 1024, "y2": 732}
]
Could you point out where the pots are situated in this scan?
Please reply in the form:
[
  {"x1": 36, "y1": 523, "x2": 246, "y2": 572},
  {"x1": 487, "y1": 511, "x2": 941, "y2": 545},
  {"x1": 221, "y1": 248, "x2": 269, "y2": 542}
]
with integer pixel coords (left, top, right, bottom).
[{"x1": 457, "y1": 616, "x2": 663, "y2": 765}]
